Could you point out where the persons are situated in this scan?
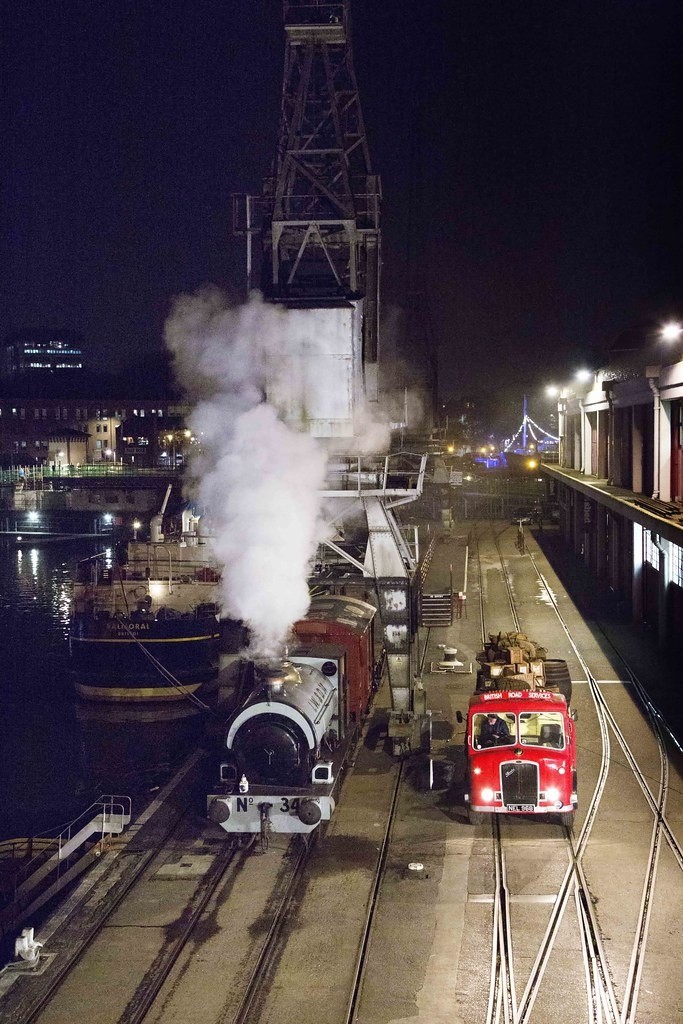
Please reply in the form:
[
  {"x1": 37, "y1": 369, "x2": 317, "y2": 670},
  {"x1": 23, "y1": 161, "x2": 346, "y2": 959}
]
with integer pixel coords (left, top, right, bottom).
[
  {"x1": 19, "y1": 462, "x2": 81, "y2": 482},
  {"x1": 479, "y1": 714, "x2": 510, "y2": 749}
]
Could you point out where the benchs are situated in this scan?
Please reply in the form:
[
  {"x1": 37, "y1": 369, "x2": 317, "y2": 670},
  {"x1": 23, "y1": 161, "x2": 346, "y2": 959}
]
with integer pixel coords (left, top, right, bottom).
[{"x1": 634, "y1": 497, "x2": 681, "y2": 517}]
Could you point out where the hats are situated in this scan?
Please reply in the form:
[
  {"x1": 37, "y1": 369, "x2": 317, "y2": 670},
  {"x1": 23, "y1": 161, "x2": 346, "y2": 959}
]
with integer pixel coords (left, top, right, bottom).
[{"x1": 488, "y1": 714, "x2": 497, "y2": 718}]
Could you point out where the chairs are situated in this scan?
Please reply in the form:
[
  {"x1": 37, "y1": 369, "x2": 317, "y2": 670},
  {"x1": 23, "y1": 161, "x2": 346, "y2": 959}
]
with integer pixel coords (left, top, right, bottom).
[{"x1": 538, "y1": 724, "x2": 563, "y2": 745}]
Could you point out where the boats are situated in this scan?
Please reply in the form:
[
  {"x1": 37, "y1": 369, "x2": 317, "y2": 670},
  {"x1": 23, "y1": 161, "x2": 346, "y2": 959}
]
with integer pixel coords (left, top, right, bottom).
[
  {"x1": 0, "y1": 838, "x2": 97, "y2": 971},
  {"x1": 69, "y1": 484, "x2": 243, "y2": 702}
]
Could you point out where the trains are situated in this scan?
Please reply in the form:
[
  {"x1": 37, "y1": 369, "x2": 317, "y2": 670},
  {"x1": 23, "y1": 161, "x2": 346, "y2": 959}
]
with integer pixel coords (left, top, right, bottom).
[{"x1": 207, "y1": 594, "x2": 386, "y2": 856}]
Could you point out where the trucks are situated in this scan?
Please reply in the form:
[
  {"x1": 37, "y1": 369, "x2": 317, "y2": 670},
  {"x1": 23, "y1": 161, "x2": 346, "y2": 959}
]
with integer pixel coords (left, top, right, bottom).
[{"x1": 456, "y1": 661, "x2": 580, "y2": 828}]
